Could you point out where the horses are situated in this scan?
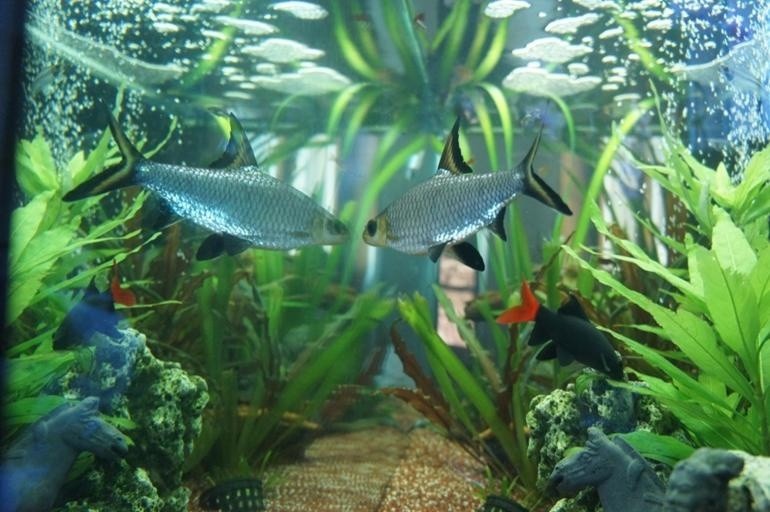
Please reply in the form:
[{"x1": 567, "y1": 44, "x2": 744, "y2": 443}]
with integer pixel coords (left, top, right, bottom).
[
  {"x1": 548, "y1": 426, "x2": 667, "y2": 512},
  {"x1": 0, "y1": 395, "x2": 136, "y2": 511}
]
